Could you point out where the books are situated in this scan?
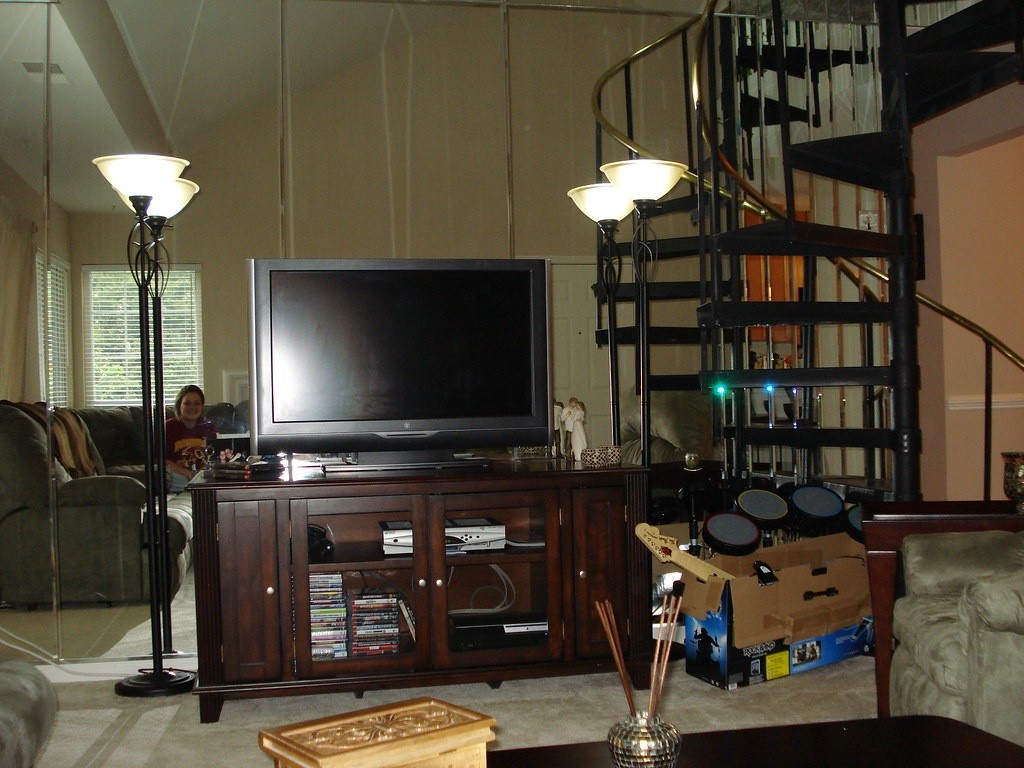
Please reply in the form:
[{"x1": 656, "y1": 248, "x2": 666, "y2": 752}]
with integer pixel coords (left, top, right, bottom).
[{"x1": 309, "y1": 572, "x2": 399, "y2": 660}]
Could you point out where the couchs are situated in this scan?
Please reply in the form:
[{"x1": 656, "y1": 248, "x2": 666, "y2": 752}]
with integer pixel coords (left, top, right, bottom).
[
  {"x1": 1, "y1": 400, "x2": 235, "y2": 607},
  {"x1": 887, "y1": 530, "x2": 1023, "y2": 747}
]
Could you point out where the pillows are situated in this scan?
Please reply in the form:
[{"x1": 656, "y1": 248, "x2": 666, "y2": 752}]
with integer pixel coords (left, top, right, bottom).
[
  {"x1": 0, "y1": 404, "x2": 73, "y2": 509},
  {"x1": 154, "y1": 403, "x2": 235, "y2": 461},
  {"x1": 72, "y1": 406, "x2": 146, "y2": 466}
]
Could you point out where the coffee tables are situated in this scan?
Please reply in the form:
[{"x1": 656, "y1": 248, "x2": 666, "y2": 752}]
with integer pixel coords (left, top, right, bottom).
[{"x1": 485, "y1": 714, "x2": 1024, "y2": 768}]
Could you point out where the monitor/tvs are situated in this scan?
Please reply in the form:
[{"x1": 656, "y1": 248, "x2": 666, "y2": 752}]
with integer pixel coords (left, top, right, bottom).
[{"x1": 246, "y1": 258, "x2": 553, "y2": 472}]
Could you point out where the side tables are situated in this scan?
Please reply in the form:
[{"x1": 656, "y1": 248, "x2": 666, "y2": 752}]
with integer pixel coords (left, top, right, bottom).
[{"x1": 859, "y1": 500, "x2": 1024, "y2": 718}]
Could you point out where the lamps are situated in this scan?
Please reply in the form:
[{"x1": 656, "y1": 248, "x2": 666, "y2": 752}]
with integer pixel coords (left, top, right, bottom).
[
  {"x1": 565, "y1": 182, "x2": 633, "y2": 447},
  {"x1": 92, "y1": 152, "x2": 195, "y2": 698},
  {"x1": 600, "y1": 159, "x2": 687, "y2": 469},
  {"x1": 129, "y1": 179, "x2": 205, "y2": 657}
]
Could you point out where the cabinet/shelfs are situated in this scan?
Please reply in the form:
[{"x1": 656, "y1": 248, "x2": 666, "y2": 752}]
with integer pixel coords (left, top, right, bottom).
[{"x1": 184, "y1": 455, "x2": 655, "y2": 724}]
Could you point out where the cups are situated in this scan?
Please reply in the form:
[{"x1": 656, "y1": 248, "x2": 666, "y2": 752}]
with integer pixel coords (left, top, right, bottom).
[{"x1": 580, "y1": 445, "x2": 621, "y2": 468}]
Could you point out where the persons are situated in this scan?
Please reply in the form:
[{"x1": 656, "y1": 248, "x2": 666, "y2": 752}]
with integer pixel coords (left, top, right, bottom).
[
  {"x1": 551, "y1": 397, "x2": 588, "y2": 459},
  {"x1": 694, "y1": 627, "x2": 718, "y2": 659},
  {"x1": 166, "y1": 385, "x2": 217, "y2": 493},
  {"x1": 220, "y1": 449, "x2": 245, "y2": 463}
]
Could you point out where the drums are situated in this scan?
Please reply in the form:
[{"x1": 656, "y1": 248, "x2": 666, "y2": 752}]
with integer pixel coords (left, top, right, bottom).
[
  {"x1": 730, "y1": 485, "x2": 792, "y2": 533},
  {"x1": 699, "y1": 508, "x2": 764, "y2": 557},
  {"x1": 843, "y1": 501, "x2": 870, "y2": 548},
  {"x1": 788, "y1": 483, "x2": 849, "y2": 524}
]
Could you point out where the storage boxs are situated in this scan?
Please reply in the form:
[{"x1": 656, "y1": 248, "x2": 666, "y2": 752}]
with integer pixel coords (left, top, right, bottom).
[
  {"x1": 679, "y1": 533, "x2": 871, "y2": 691},
  {"x1": 258, "y1": 694, "x2": 497, "y2": 768}
]
